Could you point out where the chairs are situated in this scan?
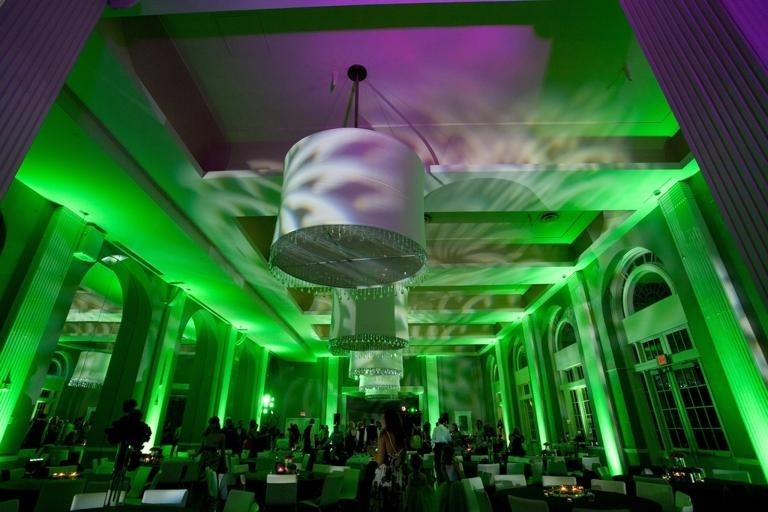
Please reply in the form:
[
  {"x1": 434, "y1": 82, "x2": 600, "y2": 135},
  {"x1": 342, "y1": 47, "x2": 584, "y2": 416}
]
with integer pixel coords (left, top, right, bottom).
[{"x1": 0, "y1": 447, "x2": 693, "y2": 512}]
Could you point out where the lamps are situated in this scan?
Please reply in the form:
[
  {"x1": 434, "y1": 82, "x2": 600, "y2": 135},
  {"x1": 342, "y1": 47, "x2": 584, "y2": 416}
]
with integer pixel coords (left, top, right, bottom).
[
  {"x1": 67, "y1": 351, "x2": 112, "y2": 391},
  {"x1": 266, "y1": 64, "x2": 429, "y2": 402}
]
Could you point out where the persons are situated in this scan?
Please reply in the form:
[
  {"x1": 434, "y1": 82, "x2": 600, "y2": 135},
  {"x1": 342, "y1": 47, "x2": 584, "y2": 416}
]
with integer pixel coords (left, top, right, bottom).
[
  {"x1": 20, "y1": 399, "x2": 152, "y2": 490},
  {"x1": 164, "y1": 412, "x2": 528, "y2": 511}
]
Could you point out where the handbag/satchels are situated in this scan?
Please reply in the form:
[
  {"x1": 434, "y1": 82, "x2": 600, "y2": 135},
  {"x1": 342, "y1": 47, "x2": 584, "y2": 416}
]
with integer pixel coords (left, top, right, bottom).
[{"x1": 369, "y1": 428, "x2": 405, "y2": 497}]
